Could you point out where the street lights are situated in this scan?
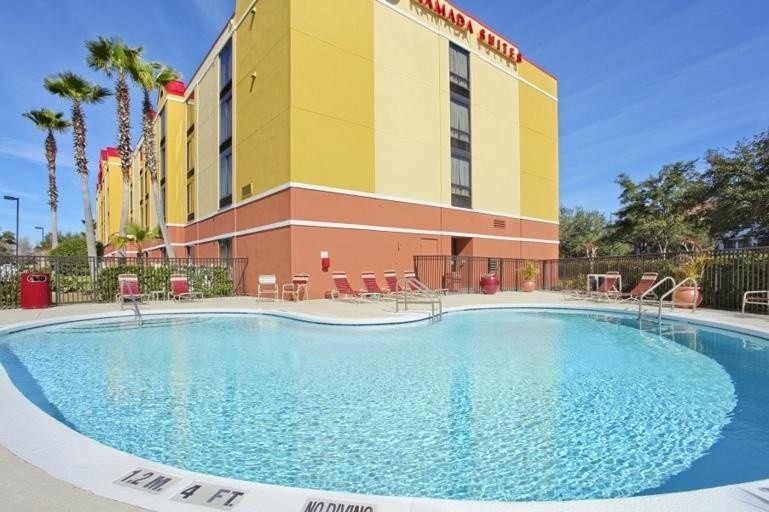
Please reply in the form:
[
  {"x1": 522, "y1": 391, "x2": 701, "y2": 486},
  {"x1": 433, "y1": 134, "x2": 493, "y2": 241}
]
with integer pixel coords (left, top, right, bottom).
[
  {"x1": 35, "y1": 226, "x2": 44, "y2": 240},
  {"x1": 4, "y1": 195, "x2": 19, "y2": 272}
]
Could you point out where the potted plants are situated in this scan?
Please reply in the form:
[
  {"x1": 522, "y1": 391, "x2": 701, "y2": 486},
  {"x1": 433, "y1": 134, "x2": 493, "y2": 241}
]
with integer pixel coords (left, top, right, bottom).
[
  {"x1": 516, "y1": 257, "x2": 540, "y2": 291},
  {"x1": 667, "y1": 243, "x2": 706, "y2": 308}
]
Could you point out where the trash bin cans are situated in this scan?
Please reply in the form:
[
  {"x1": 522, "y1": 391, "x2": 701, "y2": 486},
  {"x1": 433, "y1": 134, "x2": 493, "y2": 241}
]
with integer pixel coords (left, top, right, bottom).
[
  {"x1": 444, "y1": 271, "x2": 462, "y2": 292},
  {"x1": 21, "y1": 273, "x2": 49, "y2": 309}
]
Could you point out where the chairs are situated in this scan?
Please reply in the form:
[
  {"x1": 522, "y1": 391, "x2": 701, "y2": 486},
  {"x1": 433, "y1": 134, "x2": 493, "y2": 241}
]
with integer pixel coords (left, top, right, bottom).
[
  {"x1": 257, "y1": 271, "x2": 312, "y2": 302},
  {"x1": 741, "y1": 290, "x2": 769, "y2": 318},
  {"x1": 116, "y1": 273, "x2": 204, "y2": 303},
  {"x1": 562, "y1": 271, "x2": 658, "y2": 304},
  {"x1": 328, "y1": 271, "x2": 449, "y2": 303}
]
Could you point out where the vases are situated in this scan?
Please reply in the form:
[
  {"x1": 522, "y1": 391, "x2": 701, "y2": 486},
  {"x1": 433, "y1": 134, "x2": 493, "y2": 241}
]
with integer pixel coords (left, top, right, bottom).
[{"x1": 480, "y1": 273, "x2": 500, "y2": 294}]
